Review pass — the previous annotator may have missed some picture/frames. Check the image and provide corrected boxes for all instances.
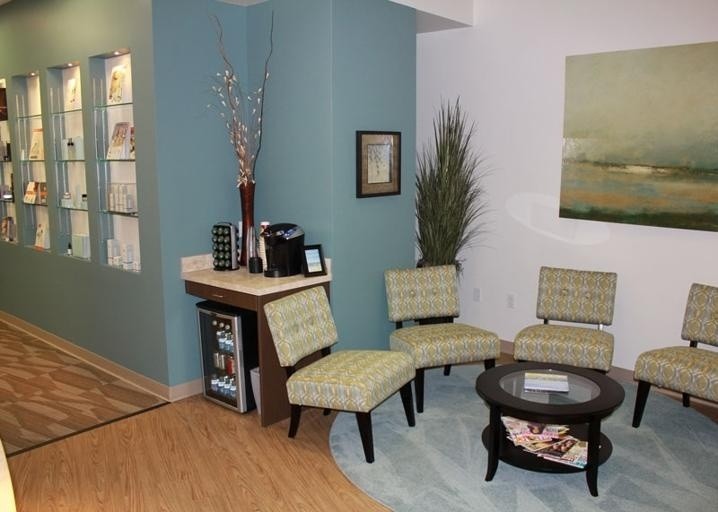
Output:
[301,244,327,277]
[357,130,401,198]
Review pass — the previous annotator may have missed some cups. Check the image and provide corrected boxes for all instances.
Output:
[248,257,263,273]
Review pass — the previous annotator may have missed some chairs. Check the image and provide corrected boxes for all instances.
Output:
[383,266,501,413]
[631,282,718,427]
[264,284,420,462]
[514,268,617,375]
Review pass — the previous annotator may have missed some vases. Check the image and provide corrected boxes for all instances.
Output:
[239,182,257,267]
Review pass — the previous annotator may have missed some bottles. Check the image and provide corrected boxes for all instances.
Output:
[218,377,224,394]
[224,379,230,396]
[211,375,218,391]
[229,335,235,354]
[224,334,232,351]
[230,382,237,399]
[218,332,226,351]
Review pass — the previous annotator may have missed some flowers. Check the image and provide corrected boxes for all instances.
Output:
[198,10,276,188]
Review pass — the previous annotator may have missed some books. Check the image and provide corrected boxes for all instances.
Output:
[501,415,589,470]
[524,372,569,393]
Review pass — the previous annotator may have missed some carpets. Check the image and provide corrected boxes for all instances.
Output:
[329,361,718,512]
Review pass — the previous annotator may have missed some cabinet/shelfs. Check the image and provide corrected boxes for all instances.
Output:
[48,59,95,263]
[1,76,20,247]
[88,49,142,272]
[183,259,333,427]
[17,68,54,254]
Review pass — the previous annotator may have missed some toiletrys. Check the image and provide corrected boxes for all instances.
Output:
[67,138,75,160]
[80,194,87,210]
[117,183,127,211]
[109,192,115,210]
[67,242,73,256]
[127,194,134,214]
[61,192,73,208]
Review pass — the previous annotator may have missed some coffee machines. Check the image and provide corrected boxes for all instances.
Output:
[262,223,304,278]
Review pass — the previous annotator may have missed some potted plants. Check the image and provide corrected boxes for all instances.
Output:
[411,103,493,326]
